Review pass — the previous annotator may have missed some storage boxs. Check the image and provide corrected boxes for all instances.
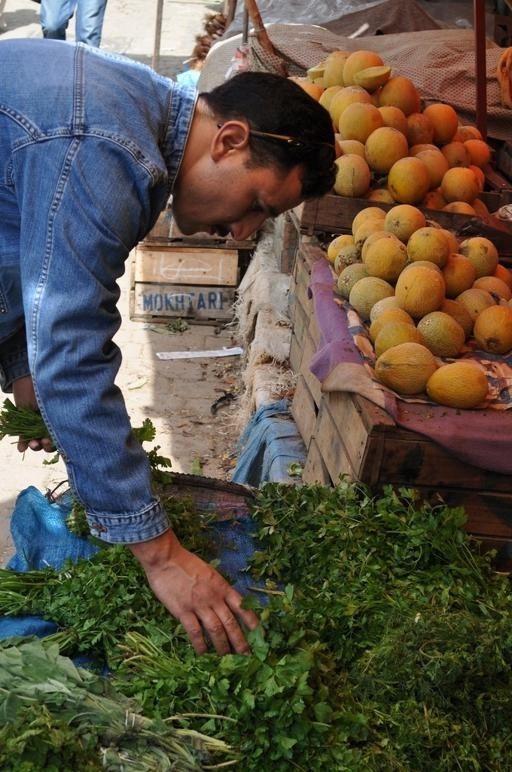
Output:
[128,210,256,326]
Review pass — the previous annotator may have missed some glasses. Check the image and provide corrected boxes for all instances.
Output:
[216,125,336,164]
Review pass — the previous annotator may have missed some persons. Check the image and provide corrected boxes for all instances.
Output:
[0,37,338,659]
[39,0,108,48]
